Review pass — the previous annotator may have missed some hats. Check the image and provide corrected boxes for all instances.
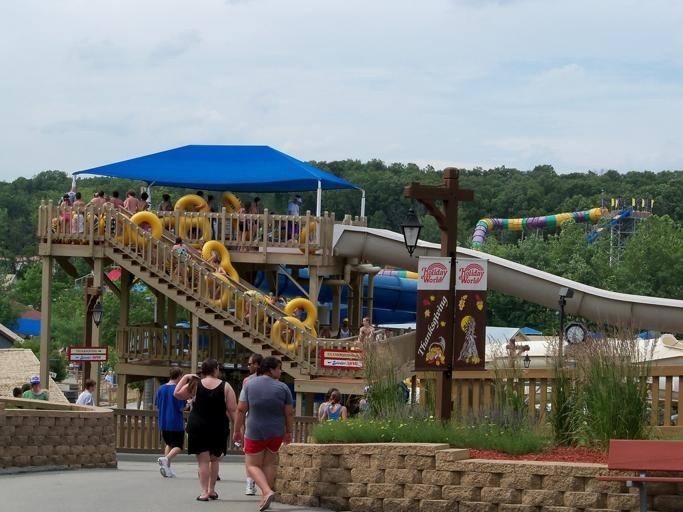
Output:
[30,375,40,384]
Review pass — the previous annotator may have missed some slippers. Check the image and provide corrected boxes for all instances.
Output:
[257,491,274,511]
[196,495,209,501]
[208,491,218,499]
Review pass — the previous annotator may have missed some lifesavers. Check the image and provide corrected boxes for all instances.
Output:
[269,298,316,351]
[222,191,241,212]
[165,254,199,284]
[174,195,212,249]
[199,240,239,308]
[298,222,316,254]
[236,290,266,325]
[50,210,174,247]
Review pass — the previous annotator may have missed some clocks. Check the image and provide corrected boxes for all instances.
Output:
[565,322,587,346]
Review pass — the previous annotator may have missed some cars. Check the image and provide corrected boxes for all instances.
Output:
[642,406,679,426]
[67,364,80,372]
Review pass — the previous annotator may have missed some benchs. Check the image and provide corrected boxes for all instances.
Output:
[596,434,681,512]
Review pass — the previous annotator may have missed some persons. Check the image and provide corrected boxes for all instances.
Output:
[23,376,50,401]
[173,359,238,502]
[13,387,22,399]
[154,367,185,478]
[240,353,264,496]
[22,383,31,394]
[233,356,293,511]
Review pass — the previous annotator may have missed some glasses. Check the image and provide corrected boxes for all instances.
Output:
[248,363,253,367]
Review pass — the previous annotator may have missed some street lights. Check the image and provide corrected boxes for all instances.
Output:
[82,284,103,395]
[555,285,573,367]
[506,338,530,397]
[601,188,604,210]
[401,164,476,428]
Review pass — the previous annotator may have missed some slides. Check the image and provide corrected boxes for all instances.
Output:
[471,208,608,252]
[330,224,682,334]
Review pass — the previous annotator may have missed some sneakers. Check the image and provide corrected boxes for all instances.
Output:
[245,477,257,496]
[158,457,176,478]
